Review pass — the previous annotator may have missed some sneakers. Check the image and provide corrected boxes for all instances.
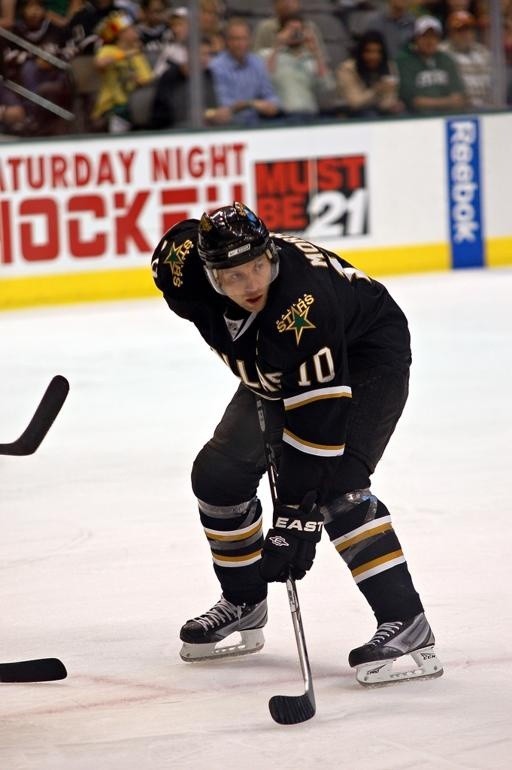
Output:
[180,592,267,644]
[348,611,435,667]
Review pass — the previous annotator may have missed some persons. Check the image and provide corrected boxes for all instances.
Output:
[152,201,445,687]
[0,0,512,143]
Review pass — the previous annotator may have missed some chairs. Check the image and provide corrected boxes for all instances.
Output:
[216,0,415,74]
[70,56,99,133]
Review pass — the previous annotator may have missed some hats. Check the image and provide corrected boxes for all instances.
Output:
[447,11,477,32]
[414,14,444,38]
[95,9,133,44]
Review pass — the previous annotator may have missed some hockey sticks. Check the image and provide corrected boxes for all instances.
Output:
[1,658,68,682]
[0,376,69,456]
[253,390,315,725]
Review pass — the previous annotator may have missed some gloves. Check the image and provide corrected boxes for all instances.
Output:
[258,490,325,582]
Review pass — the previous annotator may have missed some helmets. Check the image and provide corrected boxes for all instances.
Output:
[197,201,281,296]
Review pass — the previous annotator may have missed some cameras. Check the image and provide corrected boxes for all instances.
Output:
[293,29,303,37]
